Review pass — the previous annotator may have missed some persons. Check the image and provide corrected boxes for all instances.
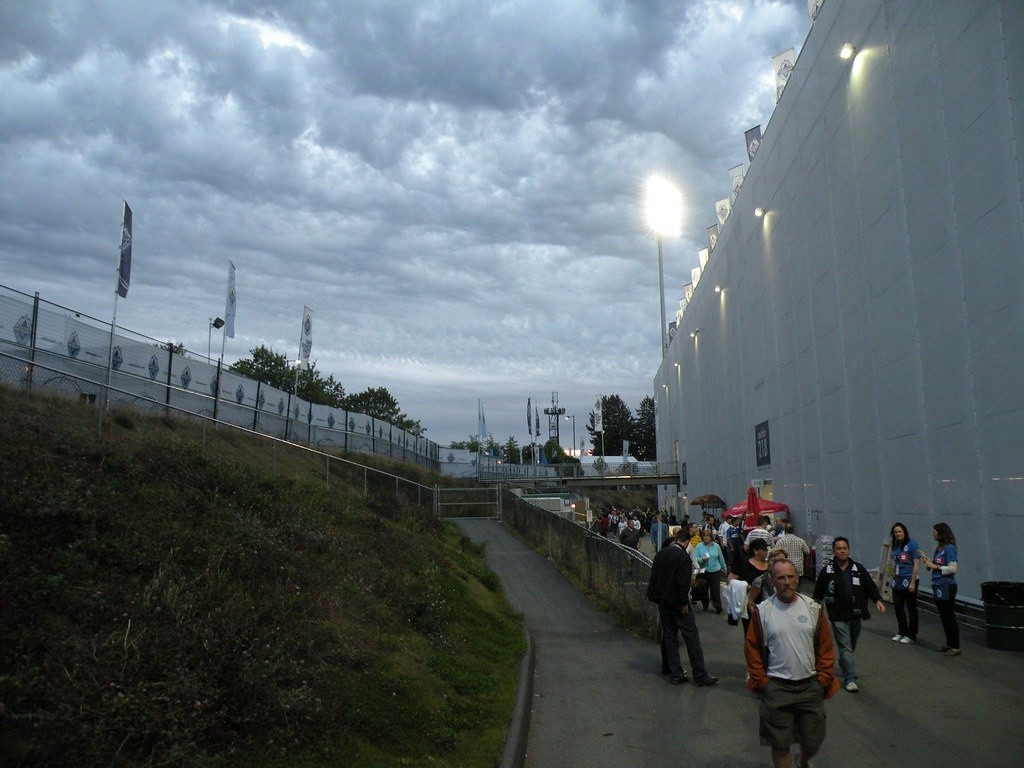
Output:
[888,522,920,644]
[597,505,655,549]
[923,522,960,656]
[652,511,810,639]
[744,558,841,768]
[646,530,720,686]
[812,536,886,691]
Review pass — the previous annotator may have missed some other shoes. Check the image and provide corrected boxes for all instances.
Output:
[891,634,903,642]
[696,674,719,688]
[794,755,810,768]
[900,635,915,644]
[845,681,859,693]
[691,600,697,604]
[671,671,689,684]
[703,605,708,611]
[715,604,724,614]
[943,648,961,656]
[935,644,952,652]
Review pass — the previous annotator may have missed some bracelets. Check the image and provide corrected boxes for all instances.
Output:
[937,565,939,569]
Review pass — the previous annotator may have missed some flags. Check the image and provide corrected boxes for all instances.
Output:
[593,395,602,431]
[526,399,541,436]
[478,400,487,438]
[117,201,133,298]
[224,263,237,339]
[301,306,313,358]
[623,440,629,462]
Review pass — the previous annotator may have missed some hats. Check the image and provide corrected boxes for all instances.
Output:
[749,538,771,548]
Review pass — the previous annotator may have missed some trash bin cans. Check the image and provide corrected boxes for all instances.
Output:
[981,581,1024,650]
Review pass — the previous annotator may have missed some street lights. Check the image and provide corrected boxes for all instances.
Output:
[565,414,576,456]
[207,316,225,365]
[600,429,606,463]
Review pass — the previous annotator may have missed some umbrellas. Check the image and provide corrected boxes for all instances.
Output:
[690,493,726,508]
[721,497,788,518]
[744,486,760,530]
